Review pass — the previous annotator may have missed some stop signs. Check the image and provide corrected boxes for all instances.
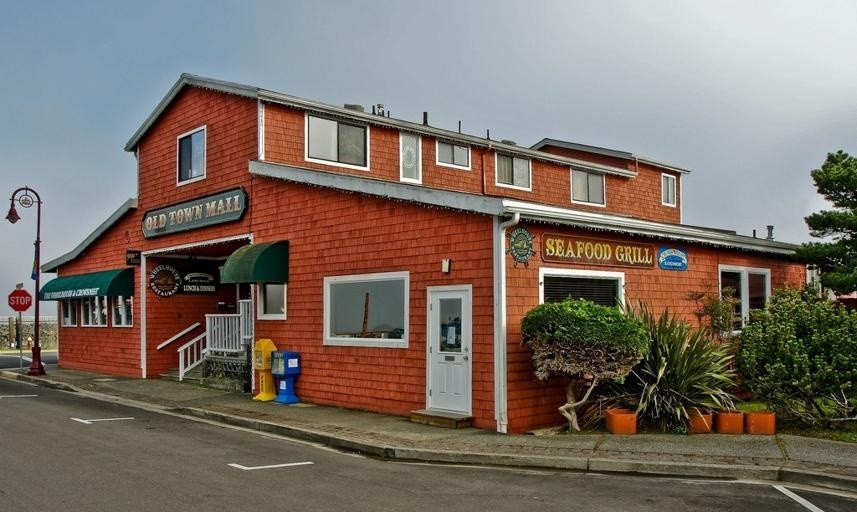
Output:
[8,289,31,312]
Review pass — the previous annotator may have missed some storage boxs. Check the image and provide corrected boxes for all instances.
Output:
[744,410,777,436]
[713,409,743,435]
[684,407,713,433]
[605,407,636,435]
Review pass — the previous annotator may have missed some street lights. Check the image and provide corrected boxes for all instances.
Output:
[4,185,47,376]
